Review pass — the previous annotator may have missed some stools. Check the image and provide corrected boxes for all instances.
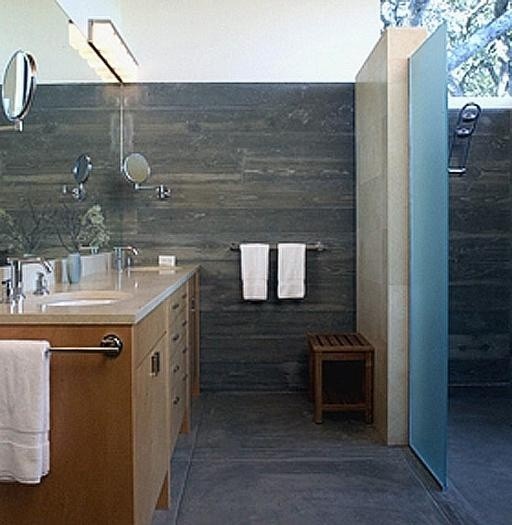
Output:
[307,331,374,425]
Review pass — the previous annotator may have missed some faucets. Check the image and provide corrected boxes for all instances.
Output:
[1,256,53,304]
[112,246,139,273]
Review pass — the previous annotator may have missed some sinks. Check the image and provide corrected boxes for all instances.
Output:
[34,288,132,308]
[125,264,182,273]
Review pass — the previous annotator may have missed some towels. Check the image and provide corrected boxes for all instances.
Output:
[241,243,269,301]
[277,242,306,299]
[0,340,50,484]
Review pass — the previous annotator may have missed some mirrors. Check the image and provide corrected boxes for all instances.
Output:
[122,151,170,200]
[0,49,37,131]
[0,0,126,292]
[73,152,92,183]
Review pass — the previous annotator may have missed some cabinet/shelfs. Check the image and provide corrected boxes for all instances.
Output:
[0,264,199,525]
